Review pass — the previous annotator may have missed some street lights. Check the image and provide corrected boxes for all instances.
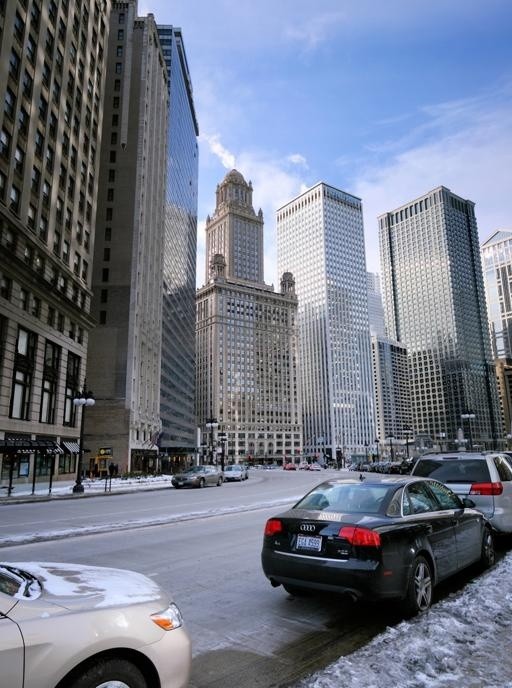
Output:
[461,404,476,451]
[72,378,95,491]
[402,424,413,458]
[205,417,219,464]
[386,429,395,460]
[374,436,380,463]
[364,440,369,461]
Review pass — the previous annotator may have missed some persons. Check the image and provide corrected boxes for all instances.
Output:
[109,462,115,479]
[114,463,118,475]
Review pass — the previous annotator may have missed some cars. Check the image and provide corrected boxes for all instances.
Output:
[283,461,321,470]
[0,560,190,688]
[262,470,505,611]
[408,450,512,542]
[171,464,224,488]
[223,464,248,481]
[348,456,415,475]
